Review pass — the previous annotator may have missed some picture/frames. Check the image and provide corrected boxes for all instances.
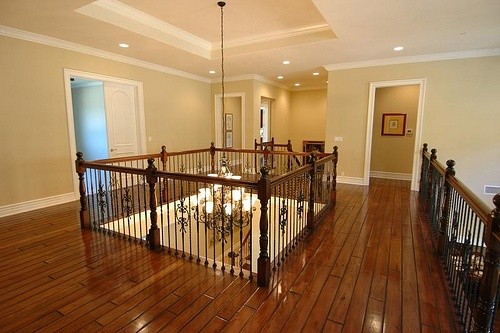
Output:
[381,112,407,137]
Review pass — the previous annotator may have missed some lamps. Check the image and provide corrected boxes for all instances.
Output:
[192,0,259,240]
